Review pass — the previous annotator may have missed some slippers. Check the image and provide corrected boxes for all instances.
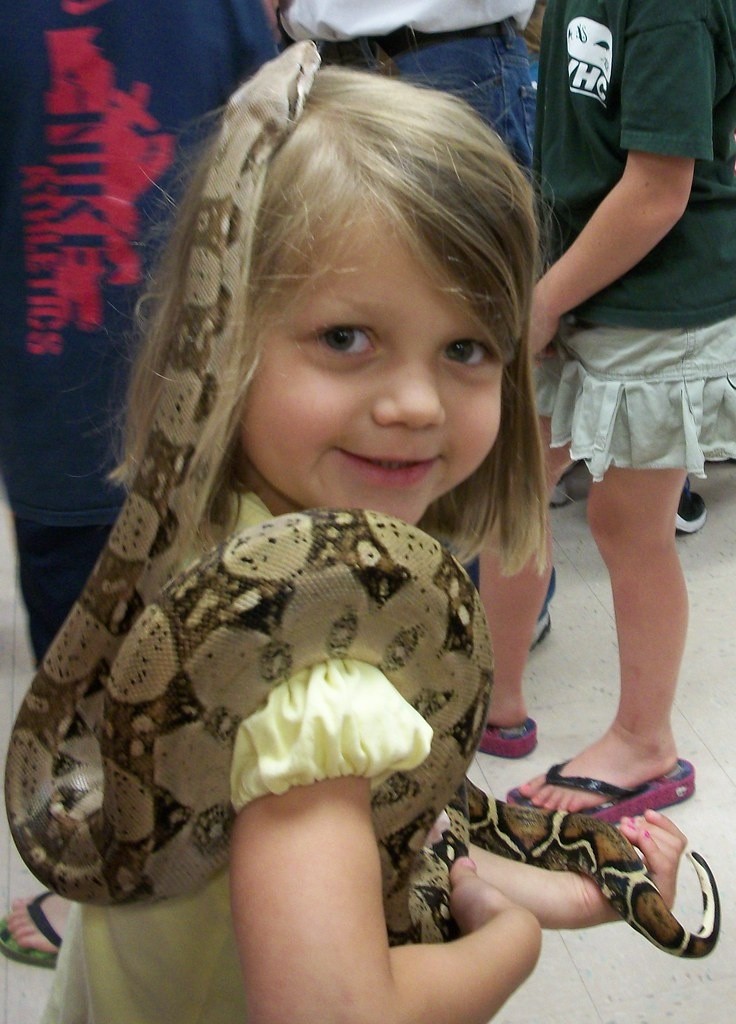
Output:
[0,891,63,969]
[507,758,697,825]
[478,717,538,759]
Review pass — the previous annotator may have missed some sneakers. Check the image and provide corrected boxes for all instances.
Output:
[528,565,556,651]
[675,476,707,533]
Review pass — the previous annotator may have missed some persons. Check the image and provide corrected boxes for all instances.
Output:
[44,40,691,1024]
[275,0,557,651]
[476,0,736,824]
[0,0,279,671]
